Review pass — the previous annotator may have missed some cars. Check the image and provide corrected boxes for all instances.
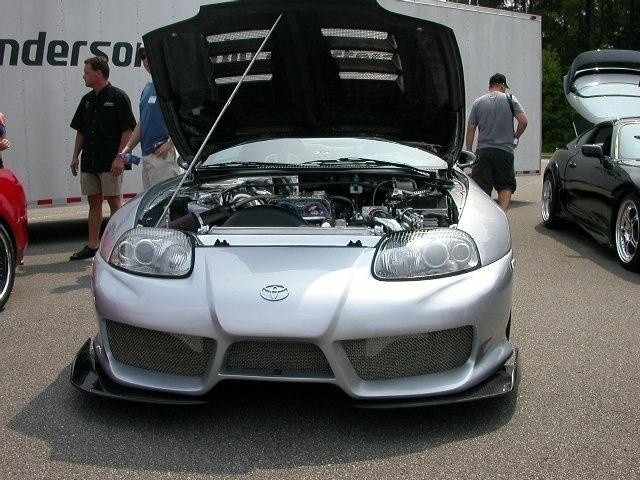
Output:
[0,167,30,311]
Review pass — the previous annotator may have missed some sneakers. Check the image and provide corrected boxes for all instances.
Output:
[70,245,98,261]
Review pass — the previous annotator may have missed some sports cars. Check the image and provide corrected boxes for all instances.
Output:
[68,0,522,414]
[541,47,640,271]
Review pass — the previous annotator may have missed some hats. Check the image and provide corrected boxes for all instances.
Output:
[490,73,510,89]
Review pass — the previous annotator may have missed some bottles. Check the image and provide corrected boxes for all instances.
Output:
[116,152,144,168]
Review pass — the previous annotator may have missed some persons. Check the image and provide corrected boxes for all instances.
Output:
[69,56,136,262]
[465,73,528,213]
[0,112,12,168]
[117,43,187,193]
